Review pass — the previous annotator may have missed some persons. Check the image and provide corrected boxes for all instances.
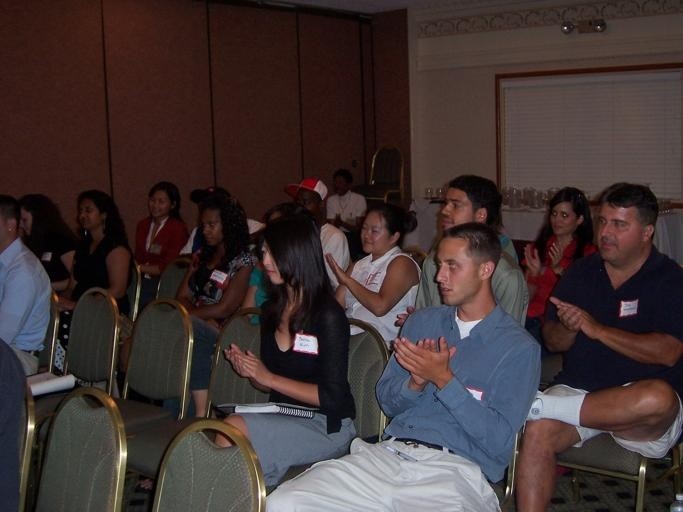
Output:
[324,170,368,264]
[134,180,190,318]
[285,176,350,294]
[213,213,357,486]
[325,200,426,356]
[520,186,599,357]
[394,174,529,331]
[239,203,289,328]
[177,186,267,267]
[163,197,260,418]
[512,182,683,512]
[266,222,541,512]
[19,192,78,292]
[52,190,133,394]
[0,193,51,377]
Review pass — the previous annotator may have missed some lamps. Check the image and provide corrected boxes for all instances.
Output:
[561,19,607,34]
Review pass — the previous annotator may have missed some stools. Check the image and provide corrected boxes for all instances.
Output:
[505,377,679,512]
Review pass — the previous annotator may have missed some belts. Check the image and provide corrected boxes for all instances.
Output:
[385,436,454,453]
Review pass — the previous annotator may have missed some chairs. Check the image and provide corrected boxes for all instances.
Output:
[37,386,128,512]
[25,290,60,378]
[266,317,389,496]
[156,258,192,300]
[17,382,36,512]
[153,419,266,512]
[33,287,120,429]
[37,298,194,477]
[127,258,142,322]
[124,307,270,480]
[365,143,404,204]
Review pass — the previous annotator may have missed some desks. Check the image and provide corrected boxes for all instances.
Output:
[402,198,672,260]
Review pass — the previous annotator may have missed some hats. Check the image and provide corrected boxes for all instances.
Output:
[190,186,230,203]
[285,177,328,200]
